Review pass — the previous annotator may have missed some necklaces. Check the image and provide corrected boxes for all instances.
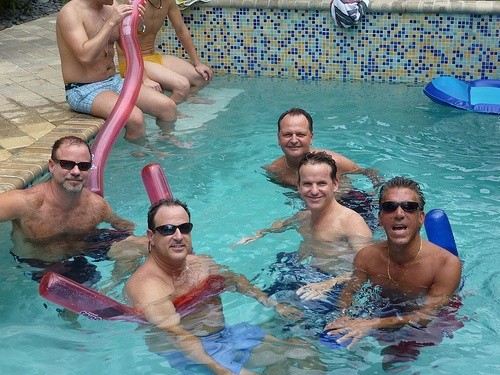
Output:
[387,238,422,285]
[148,0,162,9]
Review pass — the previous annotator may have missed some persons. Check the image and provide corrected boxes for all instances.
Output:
[115,0,215,102]
[294,152,372,236]
[264,109,357,183]
[352,176,462,297]
[123,198,217,299]
[0,136,147,246]
[56,0,191,140]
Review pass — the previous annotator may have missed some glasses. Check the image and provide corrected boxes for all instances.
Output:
[152,223,193,236]
[53,159,91,171]
[379,201,422,212]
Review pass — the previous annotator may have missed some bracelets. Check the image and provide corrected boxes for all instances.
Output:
[138,24,146,33]
